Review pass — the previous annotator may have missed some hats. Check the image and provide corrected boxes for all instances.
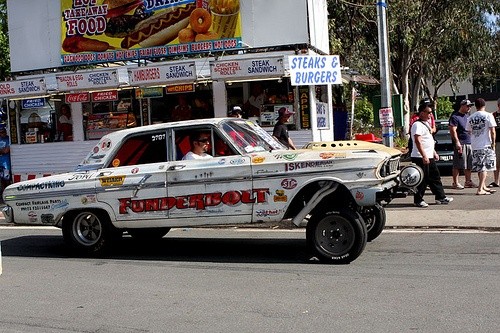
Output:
[420,98,430,104]
[461,99,474,106]
[232,106,242,113]
[275,106,296,121]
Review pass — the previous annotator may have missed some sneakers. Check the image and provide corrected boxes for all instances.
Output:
[414,201,429,208]
[464,181,479,188]
[436,197,453,204]
[452,182,464,189]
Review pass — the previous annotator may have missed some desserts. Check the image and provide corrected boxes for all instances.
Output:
[105,11,154,38]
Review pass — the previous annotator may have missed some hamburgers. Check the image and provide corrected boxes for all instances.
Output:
[101,0,144,17]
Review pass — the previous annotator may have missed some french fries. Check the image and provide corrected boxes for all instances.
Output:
[208,0,241,40]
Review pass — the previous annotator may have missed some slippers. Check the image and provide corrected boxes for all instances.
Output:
[476,190,491,195]
[487,189,497,193]
[488,182,499,187]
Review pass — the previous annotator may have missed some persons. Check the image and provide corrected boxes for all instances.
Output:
[182,132,211,161]
[172,95,210,122]
[465,98,497,195]
[272,107,296,150]
[410,103,453,208]
[487,98,500,188]
[0,127,11,192]
[232,106,242,118]
[55,104,72,142]
[409,98,437,135]
[448,99,479,190]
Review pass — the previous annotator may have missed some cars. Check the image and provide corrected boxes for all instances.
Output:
[407,119,466,176]
[4,118,425,266]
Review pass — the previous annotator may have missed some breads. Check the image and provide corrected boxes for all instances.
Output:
[179,29,220,44]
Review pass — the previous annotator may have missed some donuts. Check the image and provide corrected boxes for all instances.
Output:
[189,8,212,33]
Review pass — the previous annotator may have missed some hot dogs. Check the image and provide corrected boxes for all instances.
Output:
[121,4,196,48]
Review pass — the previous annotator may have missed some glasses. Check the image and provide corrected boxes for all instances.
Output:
[197,138,210,142]
[423,111,433,114]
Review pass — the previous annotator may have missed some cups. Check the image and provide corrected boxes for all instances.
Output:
[209,11,240,40]
[41,135,44,143]
